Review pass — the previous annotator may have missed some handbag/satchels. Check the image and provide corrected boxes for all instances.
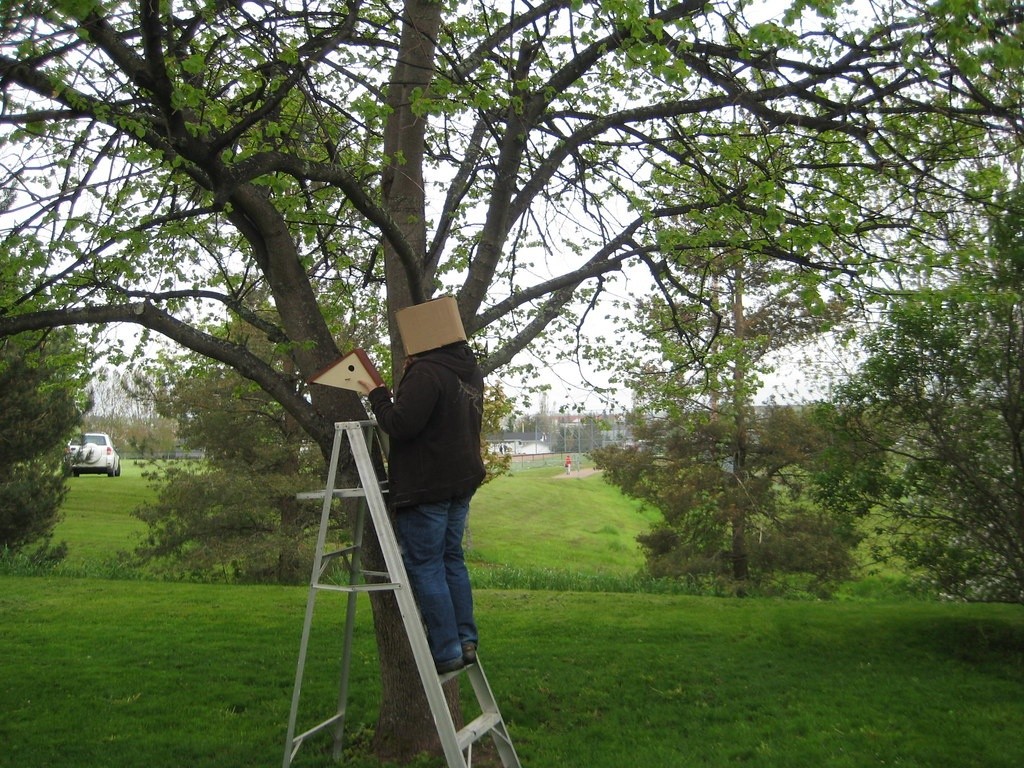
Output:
[565,464,568,467]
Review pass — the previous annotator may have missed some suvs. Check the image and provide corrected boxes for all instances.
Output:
[67,432,120,478]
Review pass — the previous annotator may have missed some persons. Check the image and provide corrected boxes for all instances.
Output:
[566,455,572,475]
[358,341,486,674]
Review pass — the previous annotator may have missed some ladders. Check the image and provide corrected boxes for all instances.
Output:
[282,419,523,768]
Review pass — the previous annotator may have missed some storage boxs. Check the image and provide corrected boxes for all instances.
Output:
[393,294,468,357]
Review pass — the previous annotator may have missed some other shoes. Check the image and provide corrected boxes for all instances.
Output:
[464,641,477,664]
[417,658,463,674]
